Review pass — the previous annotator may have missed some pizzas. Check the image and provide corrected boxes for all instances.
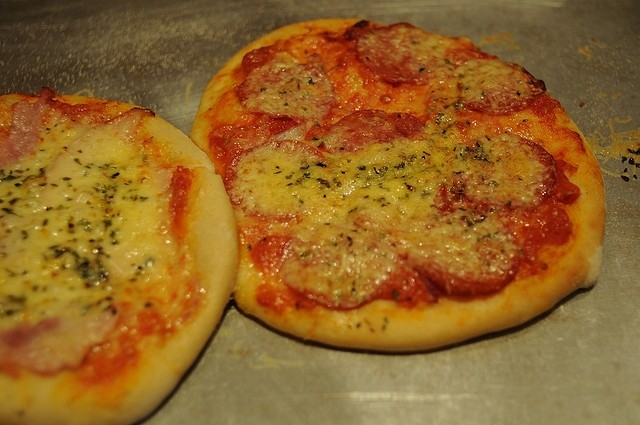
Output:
[190,16,606,357]
[0,87,239,424]
